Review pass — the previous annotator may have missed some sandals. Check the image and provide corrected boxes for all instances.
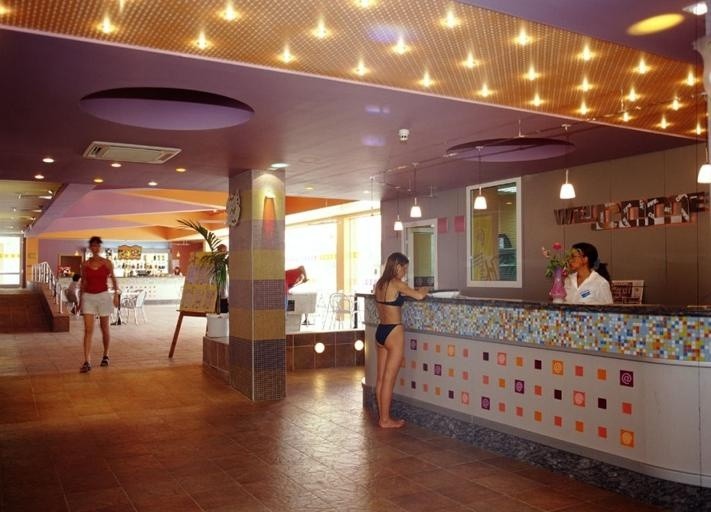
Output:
[79,362,91,373]
[100,356,108,366]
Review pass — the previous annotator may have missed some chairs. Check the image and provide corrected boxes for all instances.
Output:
[116,288,149,325]
[328,291,360,328]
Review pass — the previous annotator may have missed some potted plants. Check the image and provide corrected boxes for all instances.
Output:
[176,218,229,338]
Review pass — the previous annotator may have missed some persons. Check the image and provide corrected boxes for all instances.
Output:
[563,242,617,306]
[75,235,122,373]
[286,266,309,295]
[65,274,82,314]
[216,244,230,314]
[372,252,433,428]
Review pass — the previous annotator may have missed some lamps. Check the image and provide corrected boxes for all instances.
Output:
[394,123,576,232]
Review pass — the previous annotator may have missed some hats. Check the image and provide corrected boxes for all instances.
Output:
[88,237,103,245]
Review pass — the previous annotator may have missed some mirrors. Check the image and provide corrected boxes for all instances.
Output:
[467,176,522,288]
